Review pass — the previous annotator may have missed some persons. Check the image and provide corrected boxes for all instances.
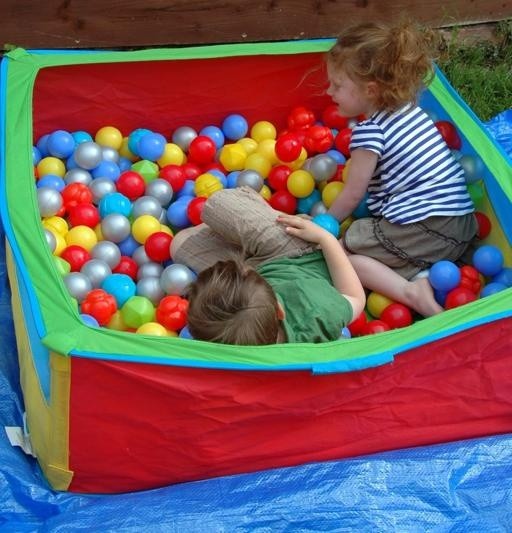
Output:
[169,186,366,345]
[295,21,479,320]
